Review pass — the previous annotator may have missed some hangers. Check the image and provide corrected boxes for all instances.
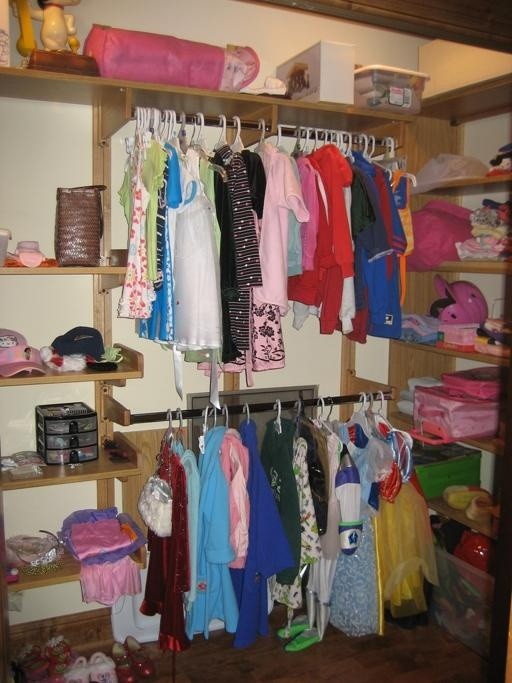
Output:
[166,391,411,480]
[124,106,417,188]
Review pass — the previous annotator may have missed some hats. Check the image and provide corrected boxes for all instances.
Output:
[0,327,47,378]
[51,326,105,356]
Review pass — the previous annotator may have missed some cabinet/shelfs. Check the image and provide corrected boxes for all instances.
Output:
[1,66,511,654]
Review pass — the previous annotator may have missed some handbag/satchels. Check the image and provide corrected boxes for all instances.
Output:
[136,433,174,539]
[54,185,108,266]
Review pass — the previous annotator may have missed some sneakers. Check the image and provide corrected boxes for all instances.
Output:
[275,614,324,654]
[88,651,119,683]
[441,483,492,510]
[463,494,494,522]
[11,635,73,683]
[63,655,91,683]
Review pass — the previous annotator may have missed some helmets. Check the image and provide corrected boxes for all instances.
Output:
[429,273,488,325]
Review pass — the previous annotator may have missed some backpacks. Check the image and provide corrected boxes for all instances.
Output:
[404,365,502,447]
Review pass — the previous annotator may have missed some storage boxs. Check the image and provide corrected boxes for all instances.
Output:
[276,40,356,105]
[432,545,496,661]
[354,64,430,115]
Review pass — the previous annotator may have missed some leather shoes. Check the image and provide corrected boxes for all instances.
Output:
[110,640,137,683]
[123,635,156,679]
